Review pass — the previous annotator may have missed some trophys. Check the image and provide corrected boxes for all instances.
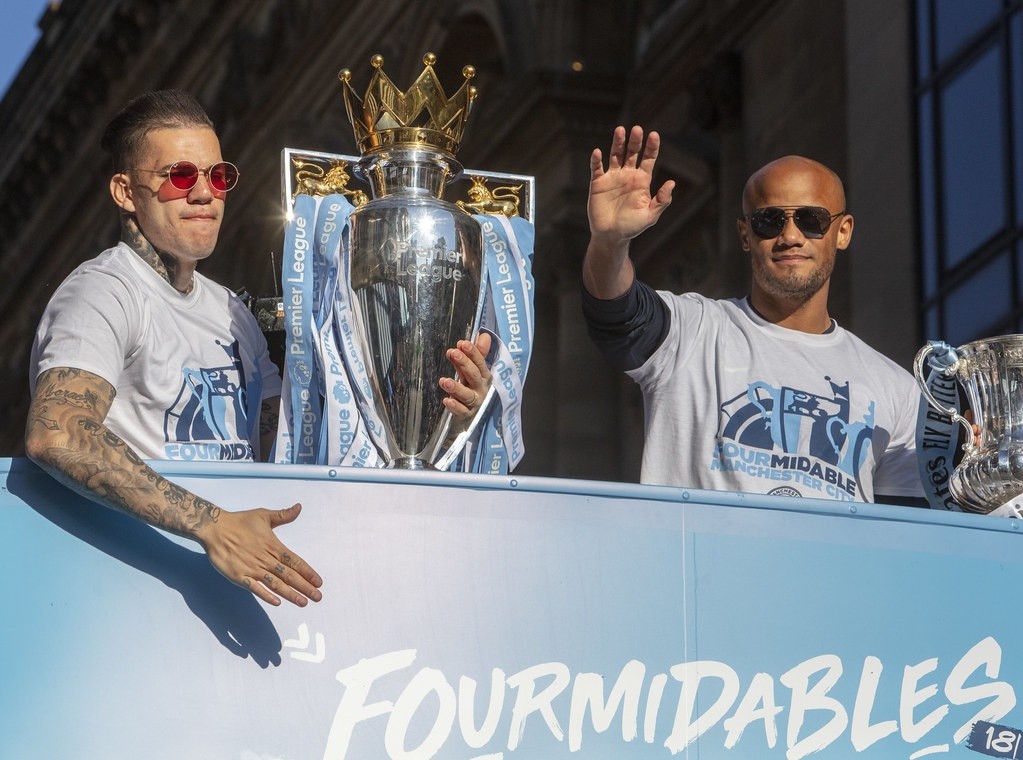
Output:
[283,51,521,470]
[913,334,1023,520]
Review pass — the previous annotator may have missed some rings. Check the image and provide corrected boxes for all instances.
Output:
[465,392,478,406]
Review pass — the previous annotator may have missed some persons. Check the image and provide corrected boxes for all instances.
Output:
[23,86,493,607]
[582,125,982,510]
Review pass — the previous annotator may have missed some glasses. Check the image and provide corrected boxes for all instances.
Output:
[741,204,845,239]
[121,160,241,193]
